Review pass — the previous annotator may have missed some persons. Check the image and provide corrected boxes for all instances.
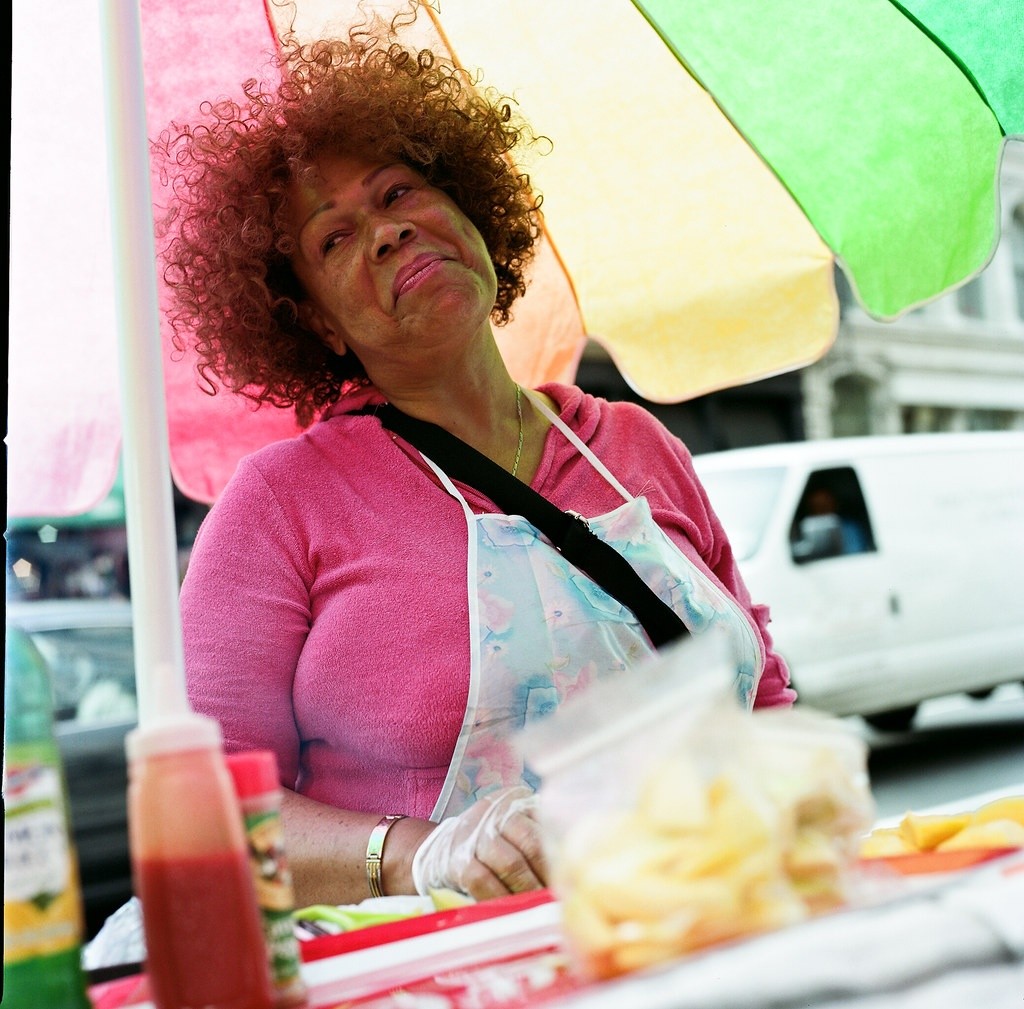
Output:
[152,23,799,905]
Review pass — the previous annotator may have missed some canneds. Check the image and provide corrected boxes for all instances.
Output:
[224,749,312,1009]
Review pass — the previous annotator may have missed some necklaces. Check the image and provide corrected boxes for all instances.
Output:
[511,383,523,476]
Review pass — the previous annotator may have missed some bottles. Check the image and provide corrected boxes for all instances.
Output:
[0,620,94,1009]
[124,711,311,1009]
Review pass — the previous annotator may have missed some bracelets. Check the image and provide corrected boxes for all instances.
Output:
[364,814,409,899]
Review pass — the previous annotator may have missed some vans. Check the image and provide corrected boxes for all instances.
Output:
[688,426,1024,745]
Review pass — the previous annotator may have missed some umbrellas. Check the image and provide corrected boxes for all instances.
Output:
[4,1,1022,730]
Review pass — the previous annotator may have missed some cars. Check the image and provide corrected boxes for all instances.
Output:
[1,603,139,944]
